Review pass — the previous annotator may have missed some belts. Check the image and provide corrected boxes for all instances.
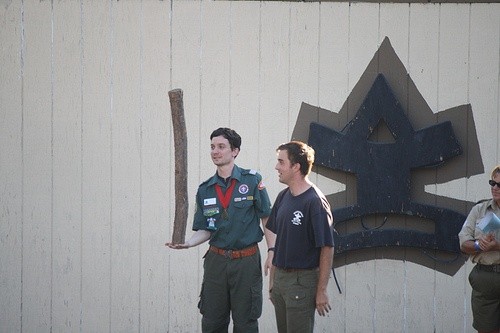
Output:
[286,267,313,271]
[209,245,258,258]
[478,263,500,273]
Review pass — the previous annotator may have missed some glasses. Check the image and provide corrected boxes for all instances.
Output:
[489,179,500,186]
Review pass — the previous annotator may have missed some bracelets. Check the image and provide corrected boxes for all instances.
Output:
[268,248,275,251]
[475,241,480,249]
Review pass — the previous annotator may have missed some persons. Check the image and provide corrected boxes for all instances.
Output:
[458,166,500,333]
[166,128,276,333]
[266,141,335,333]
